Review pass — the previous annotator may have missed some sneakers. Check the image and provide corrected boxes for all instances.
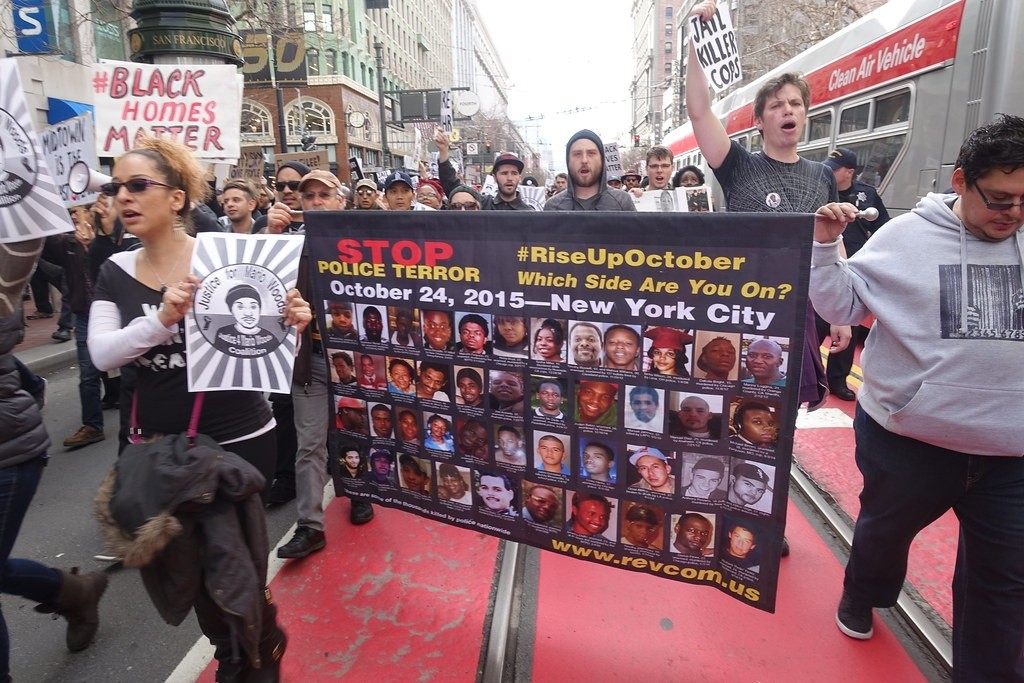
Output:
[835,587,873,639]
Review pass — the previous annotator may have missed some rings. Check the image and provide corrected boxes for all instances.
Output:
[179,282,183,289]
[305,309,306,313]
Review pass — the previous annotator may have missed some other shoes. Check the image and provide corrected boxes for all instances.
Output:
[51,326,71,340]
[26,309,53,319]
[827,383,856,401]
[62,425,107,446]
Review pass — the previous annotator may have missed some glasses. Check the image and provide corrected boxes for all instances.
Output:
[648,163,670,169]
[274,180,300,191]
[357,190,373,196]
[530,494,559,512]
[101,178,175,195]
[450,201,478,210]
[969,174,1024,210]
[626,179,637,182]
[416,193,437,198]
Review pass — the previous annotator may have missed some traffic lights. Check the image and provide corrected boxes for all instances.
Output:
[635,135,640,146]
[485,142,492,153]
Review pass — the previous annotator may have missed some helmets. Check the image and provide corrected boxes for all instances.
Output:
[369,447,394,461]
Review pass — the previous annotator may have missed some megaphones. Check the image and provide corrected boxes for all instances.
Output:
[67,161,112,194]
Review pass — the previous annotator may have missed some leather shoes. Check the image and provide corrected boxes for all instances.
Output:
[351,497,374,523]
[276,527,326,558]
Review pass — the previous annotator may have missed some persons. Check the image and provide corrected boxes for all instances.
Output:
[22,194,139,448]
[0,302,108,683]
[523,147,705,198]
[87,142,312,683]
[815,148,889,401]
[338,161,485,210]
[190,162,312,234]
[325,298,789,573]
[543,128,637,211]
[808,112,1024,683]
[685,0,853,557]
[435,127,533,209]
[261,169,373,558]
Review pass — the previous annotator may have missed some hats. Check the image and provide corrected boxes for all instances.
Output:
[620,168,641,183]
[448,186,480,202]
[275,161,311,179]
[491,154,524,174]
[644,328,694,352]
[627,505,659,524]
[298,169,342,188]
[734,463,773,492]
[820,148,857,169]
[607,176,621,182]
[521,177,538,187]
[385,173,413,190]
[399,455,430,478]
[324,300,353,314]
[355,179,376,192]
[696,340,708,373]
[338,397,366,412]
[630,447,669,466]
[456,368,483,393]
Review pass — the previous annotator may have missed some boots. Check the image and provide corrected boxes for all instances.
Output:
[33,568,108,654]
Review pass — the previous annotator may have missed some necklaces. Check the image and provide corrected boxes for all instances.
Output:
[145,256,179,291]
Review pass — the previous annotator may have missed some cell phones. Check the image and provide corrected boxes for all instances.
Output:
[289,211,305,222]
